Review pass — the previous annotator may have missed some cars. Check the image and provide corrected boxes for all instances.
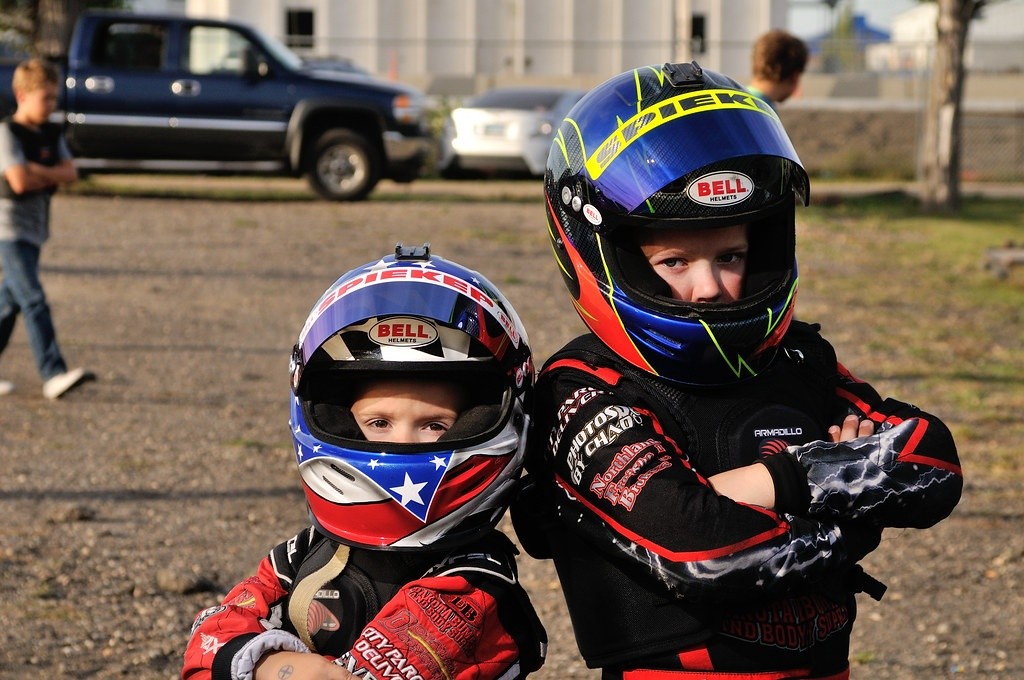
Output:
[443,87,583,180]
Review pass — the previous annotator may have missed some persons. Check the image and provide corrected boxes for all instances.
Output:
[0,57,85,401]
[507,62,963,680]
[178,242,549,680]
[745,31,810,107]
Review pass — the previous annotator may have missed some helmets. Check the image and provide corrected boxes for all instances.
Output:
[287,243,536,551]
[545,62,814,399]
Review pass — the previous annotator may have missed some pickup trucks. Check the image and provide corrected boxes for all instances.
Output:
[0,9,456,203]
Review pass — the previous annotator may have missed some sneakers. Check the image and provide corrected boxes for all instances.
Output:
[0,381,16,396]
[42,367,85,400]
